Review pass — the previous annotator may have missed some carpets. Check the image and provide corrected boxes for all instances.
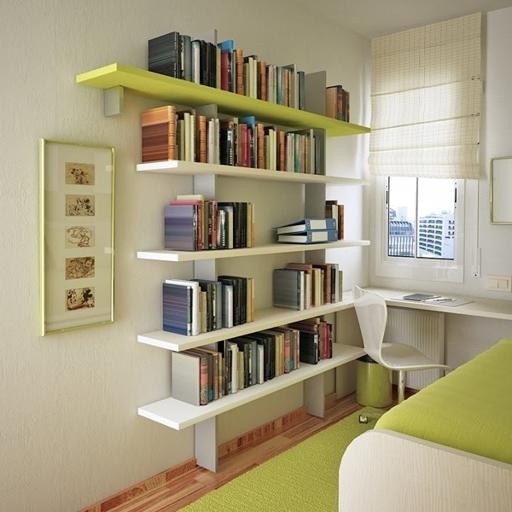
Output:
[174,406,391,512]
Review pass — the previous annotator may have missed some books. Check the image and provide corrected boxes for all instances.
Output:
[149,31,306,112]
[217,314,334,400]
[272,263,344,311]
[173,348,219,406]
[162,278,215,335]
[327,200,345,241]
[221,116,324,175]
[277,231,337,244]
[218,202,257,251]
[326,84,347,120]
[140,106,219,165]
[164,193,217,252]
[403,293,434,302]
[217,276,256,331]
[273,218,337,233]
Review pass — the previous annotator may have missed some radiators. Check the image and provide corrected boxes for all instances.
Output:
[383,307,445,392]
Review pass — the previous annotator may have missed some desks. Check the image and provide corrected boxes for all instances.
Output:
[365,286,512,321]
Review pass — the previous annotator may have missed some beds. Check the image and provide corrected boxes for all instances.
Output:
[335,334,512,512]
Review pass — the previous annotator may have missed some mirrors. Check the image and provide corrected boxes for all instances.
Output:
[490,156,511,225]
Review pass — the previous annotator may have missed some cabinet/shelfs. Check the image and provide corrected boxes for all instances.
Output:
[75,62,371,476]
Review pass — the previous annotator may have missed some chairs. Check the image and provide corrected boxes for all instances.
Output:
[349,283,449,409]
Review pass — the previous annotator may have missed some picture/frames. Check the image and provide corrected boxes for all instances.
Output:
[38,137,119,336]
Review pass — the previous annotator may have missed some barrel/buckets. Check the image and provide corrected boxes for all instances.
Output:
[354,354,393,408]
